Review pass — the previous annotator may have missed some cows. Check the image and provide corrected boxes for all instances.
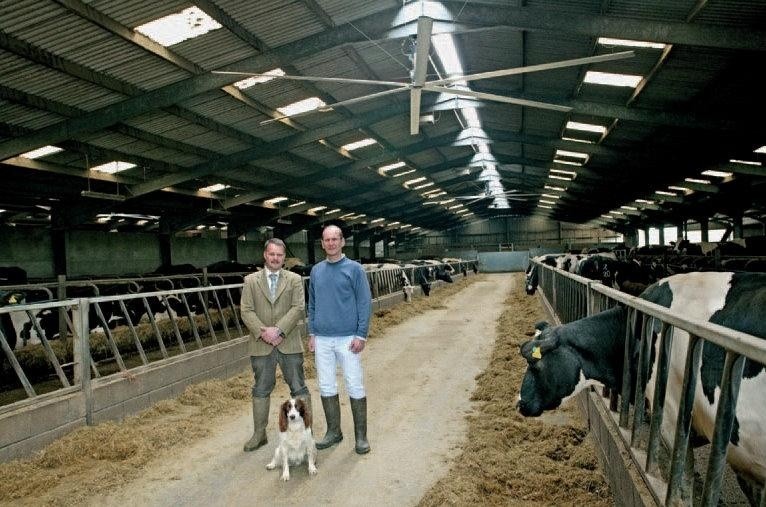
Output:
[1,256,480,355]
[516,234,765,506]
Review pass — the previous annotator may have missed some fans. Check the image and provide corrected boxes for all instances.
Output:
[209,16,637,136]
[441,178,557,209]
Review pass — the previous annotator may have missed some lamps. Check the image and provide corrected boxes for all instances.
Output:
[205,184,232,217]
[275,200,294,226]
[79,153,126,202]
[385,0,516,209]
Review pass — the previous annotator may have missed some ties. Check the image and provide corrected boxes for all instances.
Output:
[270,274,277,298]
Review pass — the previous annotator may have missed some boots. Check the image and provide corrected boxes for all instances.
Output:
[293,394,314,436]
[244,397,271,452]
[315,393,344,449]
[350,395,372,454]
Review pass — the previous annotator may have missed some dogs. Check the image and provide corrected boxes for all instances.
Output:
[264,395,319,482]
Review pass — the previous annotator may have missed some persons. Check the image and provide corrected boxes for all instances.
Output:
[241,238,312,452]
[307,225,371,454]
